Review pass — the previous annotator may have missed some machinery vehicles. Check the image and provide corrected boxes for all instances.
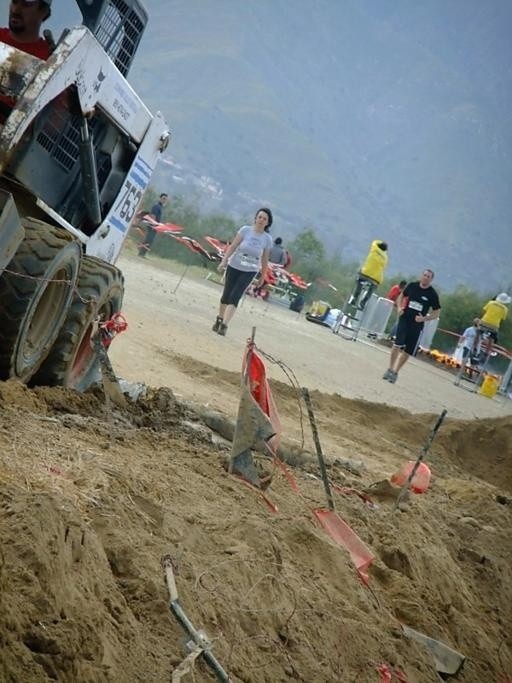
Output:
[1,1,177,397]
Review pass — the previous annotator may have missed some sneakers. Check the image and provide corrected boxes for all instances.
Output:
[360,300,365,308]
[384,368,397,384]
[213,316,228,336]
[351,300,356,305]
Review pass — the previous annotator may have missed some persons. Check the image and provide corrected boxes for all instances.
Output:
[0,0,72,135]
[473,292,512,363]
[386,280,406,303]
[282,250,292,270]
[382,270,440,384]
[212,207,272,336]
[348,240,388,310]
[457,318,481,380]
[138,193,167,259]
[257,237,286,300]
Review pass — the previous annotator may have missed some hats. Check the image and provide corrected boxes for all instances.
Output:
[496,292,512,304]
[472,318,480,325]
